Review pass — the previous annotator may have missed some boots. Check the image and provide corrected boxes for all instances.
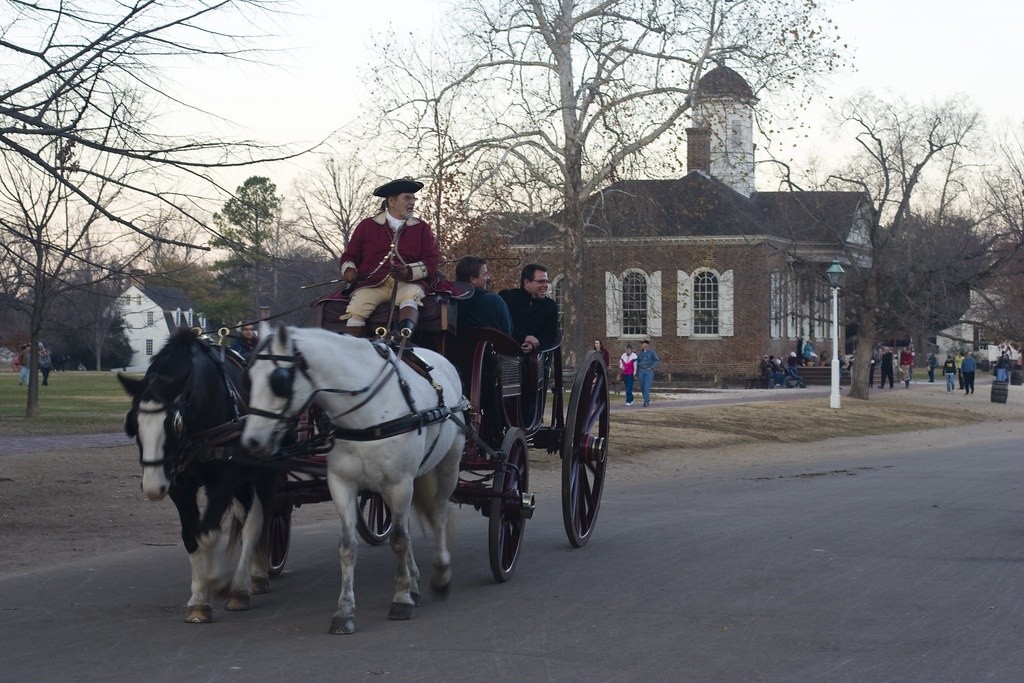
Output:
[398,306,420,341]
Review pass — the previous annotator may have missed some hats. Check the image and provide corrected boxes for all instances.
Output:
[639,339,649,344]
[791,352,796,357]
[373,179,424,198]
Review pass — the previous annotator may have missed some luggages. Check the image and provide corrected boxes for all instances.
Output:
[991,380,1009,404]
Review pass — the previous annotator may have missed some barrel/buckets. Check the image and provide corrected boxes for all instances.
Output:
[981,360,989,372]
[990,380,1008,404]
[1010,370,1023,385]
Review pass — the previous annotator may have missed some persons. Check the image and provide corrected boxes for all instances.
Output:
[498,264,558,353]
[456,255,513,337]
[18,342,53,387]
[341,180,440,342]
[636,339,659,407]
[760,353,806,389]
[620,344,638,406]
[591,339,609,409]
[839,346,1009,395]
[796,336,818,366]
[230,321,258,358]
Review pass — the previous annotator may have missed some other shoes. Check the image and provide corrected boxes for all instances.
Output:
[971,388,975,394]
[625,400,634,407]
[878,385,883,389]
[643,402,649,407]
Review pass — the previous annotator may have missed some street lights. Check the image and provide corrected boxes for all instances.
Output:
[826,256,846,409]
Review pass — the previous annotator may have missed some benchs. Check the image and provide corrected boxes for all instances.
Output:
[526,328,565,428]
[744,378,768,388]
[315,294,458,357]
[461,326,523,431]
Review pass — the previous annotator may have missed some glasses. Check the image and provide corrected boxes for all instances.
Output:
[533,279,550,284]
[402,197,419,202]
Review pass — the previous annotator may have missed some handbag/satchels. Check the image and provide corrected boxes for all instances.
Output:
[926,364,931,373]
[616,373,624,381]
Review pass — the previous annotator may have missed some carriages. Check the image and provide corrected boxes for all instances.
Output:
[114,281,610,636]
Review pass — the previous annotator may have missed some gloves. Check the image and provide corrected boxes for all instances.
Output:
[345,268,358,284]
[390,263,413,282]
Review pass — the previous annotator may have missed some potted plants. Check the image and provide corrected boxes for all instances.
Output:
[819,351,829,366]
[805,357,815,367]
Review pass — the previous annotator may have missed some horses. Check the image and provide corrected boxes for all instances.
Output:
[116,321,466,636]
